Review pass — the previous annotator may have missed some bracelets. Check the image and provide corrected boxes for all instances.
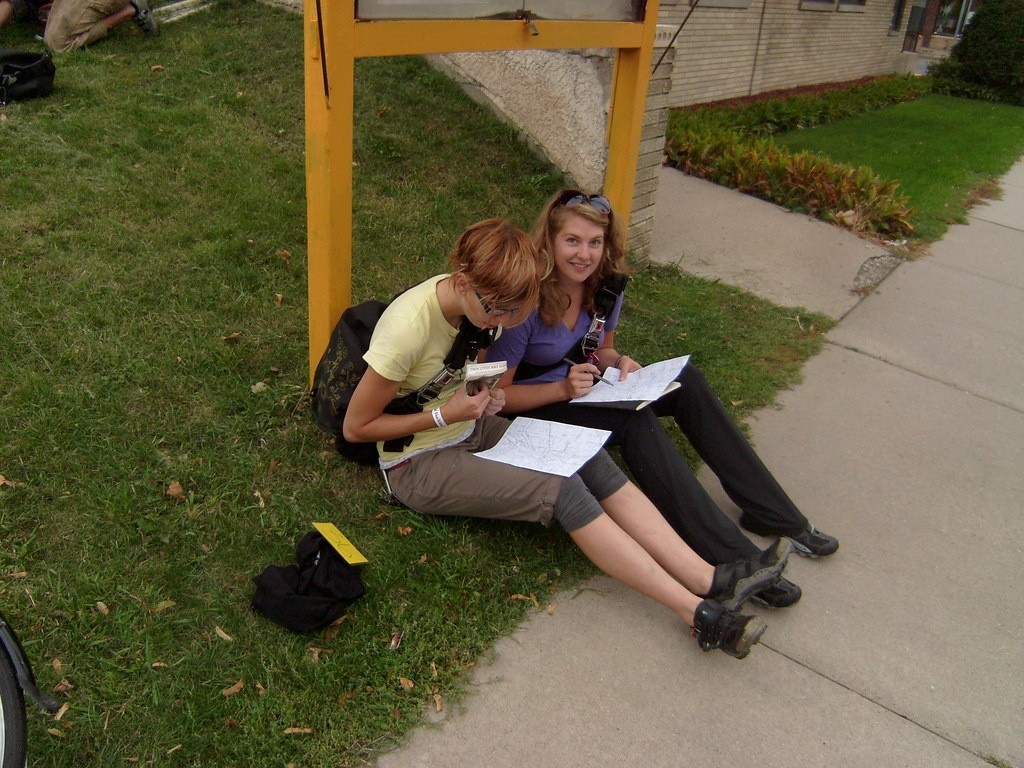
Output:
[431,407,448,429]
[615,355,630,368]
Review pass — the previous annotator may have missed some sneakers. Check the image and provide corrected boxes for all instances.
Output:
[747,576,801,611]
[131,0,159,37]
[689,600,769,659]
[712,535,792,610]
[781,522,840,556]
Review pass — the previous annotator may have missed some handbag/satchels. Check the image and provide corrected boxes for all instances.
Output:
[305,285,479,435]
[0,50,55,106]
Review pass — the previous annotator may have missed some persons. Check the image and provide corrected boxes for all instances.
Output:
[342,216,792,660]
[485,187,839,611]
[0,0,161,56]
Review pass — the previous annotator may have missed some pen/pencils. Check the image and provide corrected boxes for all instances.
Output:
[564,357,614,387]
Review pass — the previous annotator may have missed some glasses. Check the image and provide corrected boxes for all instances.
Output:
[557,190,612,216]
[469,283,522,320]
